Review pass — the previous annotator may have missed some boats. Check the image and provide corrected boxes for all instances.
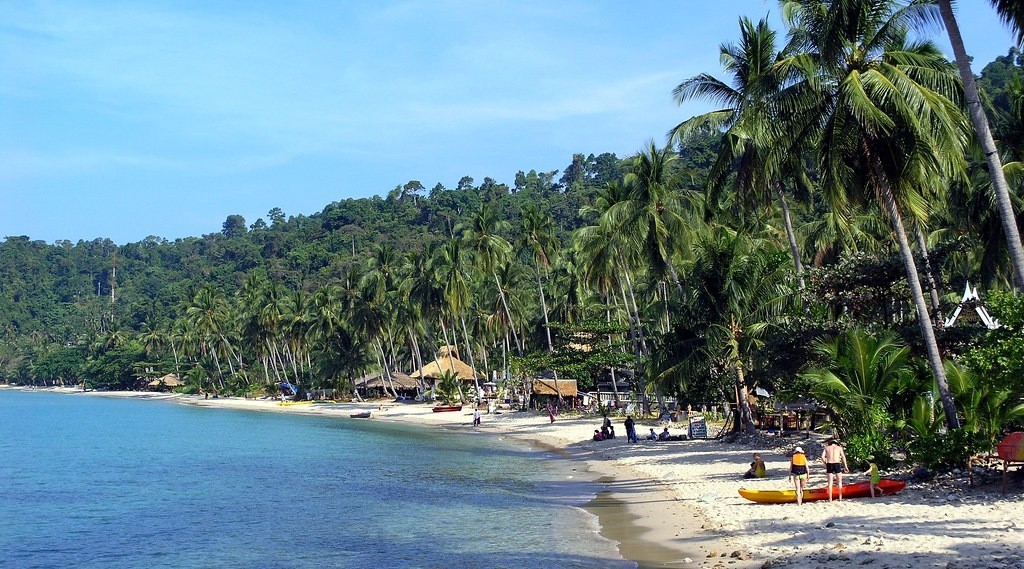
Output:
[350,412,371,418]
[431,406,462,412]
[278,400,336,407]
[737,478,906,503]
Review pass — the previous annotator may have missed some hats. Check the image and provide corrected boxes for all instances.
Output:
[866,455,875,461]
[793,447,804,453]
[828,439,840,445]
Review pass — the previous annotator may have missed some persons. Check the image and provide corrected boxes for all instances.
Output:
[647,428,669,441]
[624,415,635,443]
[789,447,809,508]
[473,409,480,426]
[744,453,765,479]
[822,440,849,502]
[862,455,883,498]
[593,413,616,440]
